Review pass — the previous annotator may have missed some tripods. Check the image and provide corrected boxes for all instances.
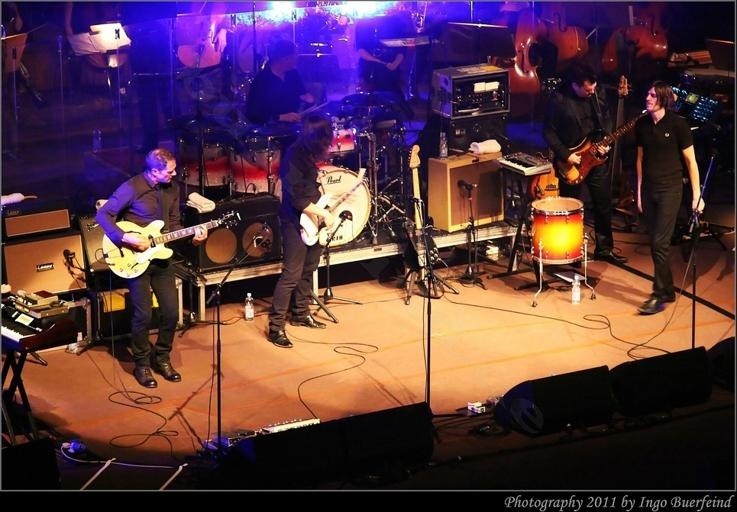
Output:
[313,219,363,315]
[2,44,145,164]
[178,270,223,338]
[353,109,413,244]
[443,190,487,290]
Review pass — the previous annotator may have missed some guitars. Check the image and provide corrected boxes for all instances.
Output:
[401,144,440,272]
[102,210,242,280]
[553,107,650,186]
[299,182,362,246]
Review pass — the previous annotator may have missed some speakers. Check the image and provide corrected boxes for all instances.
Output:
[204,420,348,489]
[340,402,434,489]
[73,210,129,290]
[2,230,88,298]
[494,366,611,439]
[609,347,712,417]
[708,337,735,393]
[4,208,72,240]
[428,151,505,233]
[192,191,282,274]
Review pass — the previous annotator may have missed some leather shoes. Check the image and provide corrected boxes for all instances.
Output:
[155,360,181,382]
[594,249,628,263]
[637,298,663,314]
[291,314,326,329]
[268,330,293,348]
[644,292,676,304]
[134,368,157,388]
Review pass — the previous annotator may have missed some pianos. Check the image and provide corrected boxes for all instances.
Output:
[495,151,551,176]
[0,303,56,353]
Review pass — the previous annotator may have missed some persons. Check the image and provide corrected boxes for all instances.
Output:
[1,1,685,134]
[93,148,208,390]
[264,113,334,348]
[545,67,630,266]
[635,78,704,314]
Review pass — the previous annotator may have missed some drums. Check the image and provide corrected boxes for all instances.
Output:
[274,158,372,248]
[172,111,360,194]
[530,197,584,266]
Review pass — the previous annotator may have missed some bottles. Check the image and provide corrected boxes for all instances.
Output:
[76,332,83,347]
[244,293,254,321]
